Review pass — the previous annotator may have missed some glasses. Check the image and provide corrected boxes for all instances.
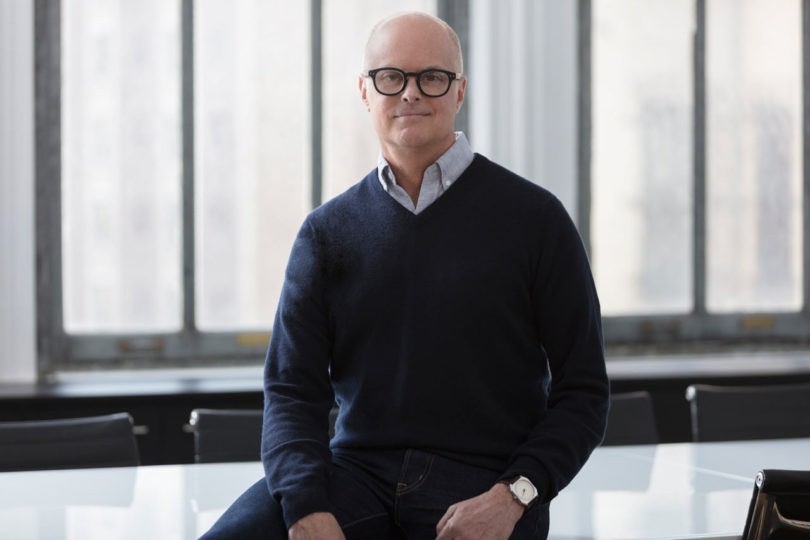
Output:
[362,66,462,98]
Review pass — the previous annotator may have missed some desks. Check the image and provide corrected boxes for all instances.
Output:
[0,347,810,420]
[0,438,810,540]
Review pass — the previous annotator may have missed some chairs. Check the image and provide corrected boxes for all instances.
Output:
[190,409,264,462]
[601,392,658,446]
[686,384,810,442]
[0,412,142,470]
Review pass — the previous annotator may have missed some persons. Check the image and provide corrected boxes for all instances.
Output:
[198,13,610,540]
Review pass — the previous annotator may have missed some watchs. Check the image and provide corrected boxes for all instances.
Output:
[497,475,539,511]
